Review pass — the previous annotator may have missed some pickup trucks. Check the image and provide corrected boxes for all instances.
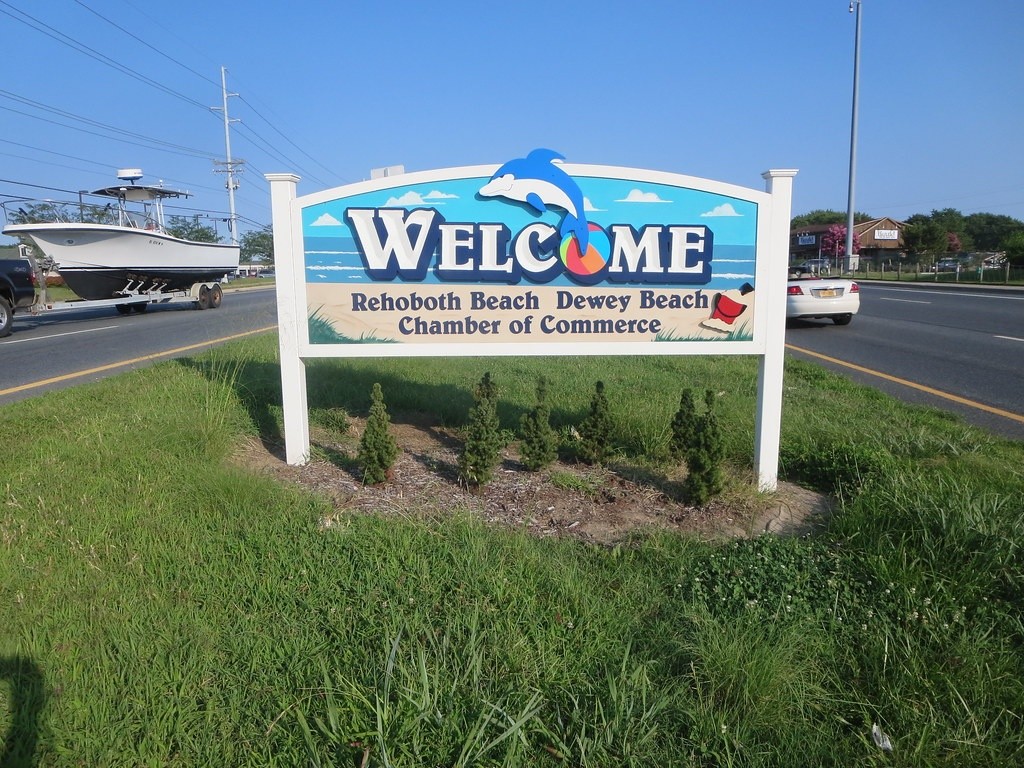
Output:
[0,256,40,338]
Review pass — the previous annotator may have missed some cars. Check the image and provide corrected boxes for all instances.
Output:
[788,258,831,276]
[255,269,275,278]
[787,266,860,324]
[955,253,978,267]
[932,259,963,271]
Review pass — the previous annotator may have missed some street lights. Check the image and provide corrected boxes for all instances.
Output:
[843,0,861,274]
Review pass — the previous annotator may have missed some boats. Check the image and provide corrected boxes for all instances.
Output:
[0,169,241,300]
